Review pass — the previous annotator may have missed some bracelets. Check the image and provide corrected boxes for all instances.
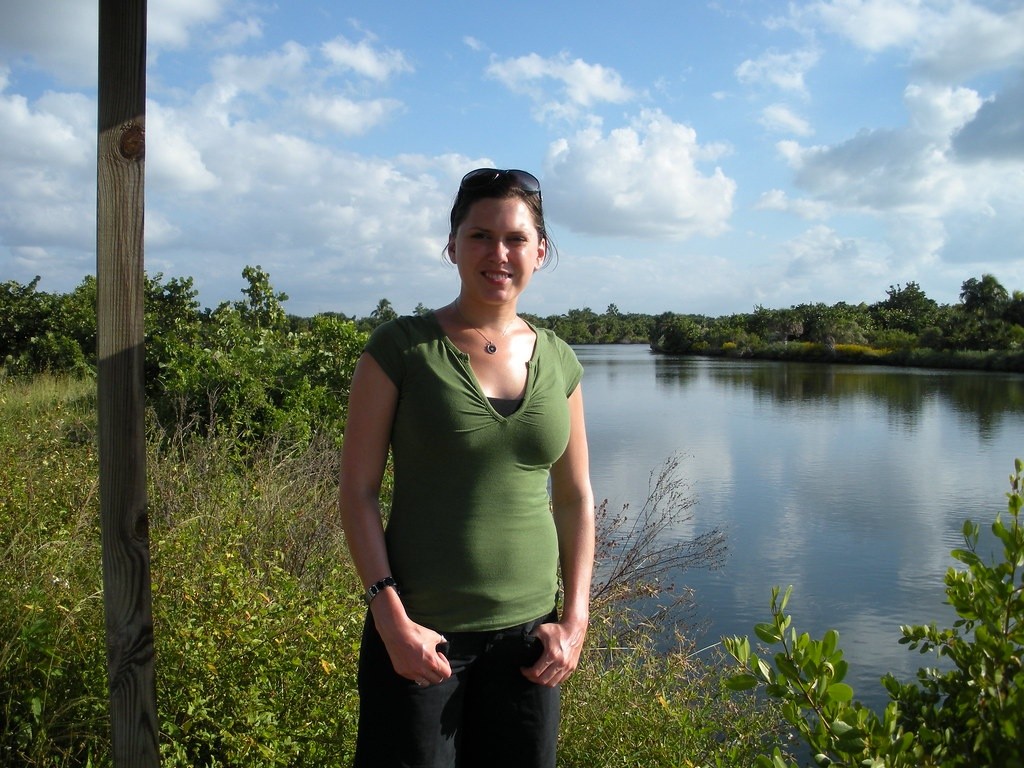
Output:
[362,576,401,605]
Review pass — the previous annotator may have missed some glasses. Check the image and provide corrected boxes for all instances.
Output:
[458,167,542,210]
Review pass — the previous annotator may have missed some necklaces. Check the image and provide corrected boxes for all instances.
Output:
[454,297,517,354]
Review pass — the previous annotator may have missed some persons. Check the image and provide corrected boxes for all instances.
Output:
[339,169,595,768]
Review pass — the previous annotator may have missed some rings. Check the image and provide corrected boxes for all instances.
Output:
[415,678,425,686]
[437,676,444,683]
